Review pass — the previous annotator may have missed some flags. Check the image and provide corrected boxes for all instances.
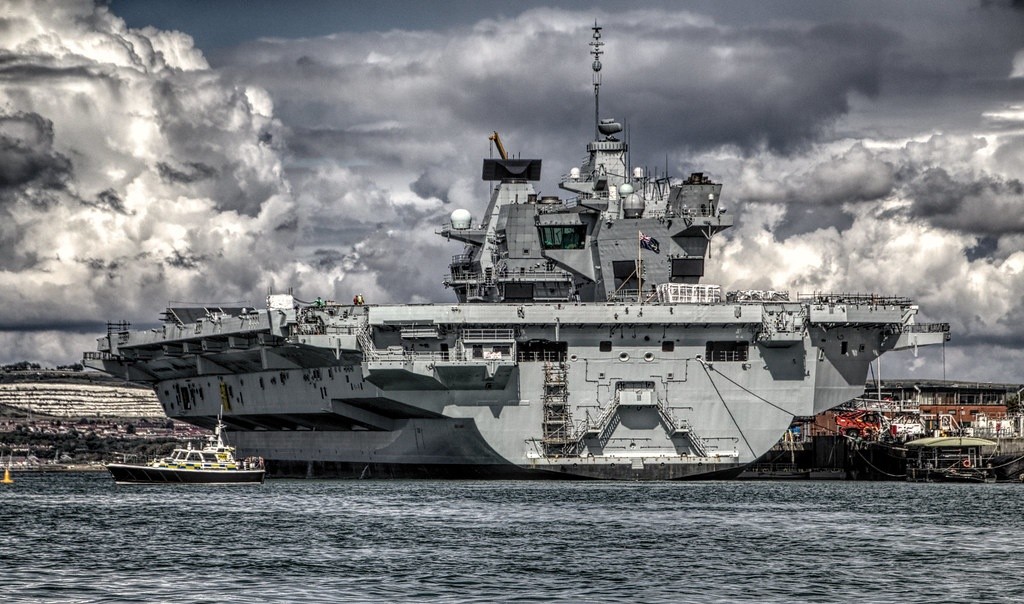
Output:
[639,231,660,254]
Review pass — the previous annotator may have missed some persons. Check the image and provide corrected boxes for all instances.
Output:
[312,296,324,304]
[240,307,247,315]
[356,292,364,304]
[352,294,358,304]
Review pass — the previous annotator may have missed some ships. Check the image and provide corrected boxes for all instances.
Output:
[81,18,919,481]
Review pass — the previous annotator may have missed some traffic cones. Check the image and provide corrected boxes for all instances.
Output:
[0,469,13,483]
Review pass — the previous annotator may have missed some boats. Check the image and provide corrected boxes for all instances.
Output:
[104,404,266,484]
